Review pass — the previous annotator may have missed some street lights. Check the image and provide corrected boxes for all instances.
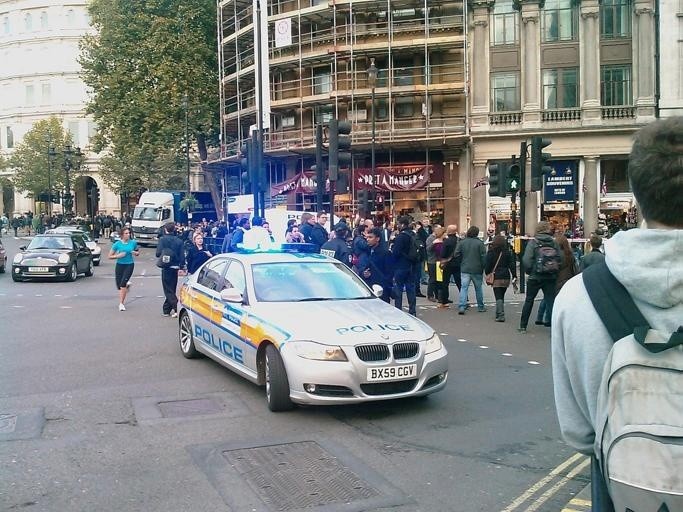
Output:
[48,143,87,219]
[363,57,380,216]
[180,89,205,199]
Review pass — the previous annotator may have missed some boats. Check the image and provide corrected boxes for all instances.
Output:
[529,135,552,192]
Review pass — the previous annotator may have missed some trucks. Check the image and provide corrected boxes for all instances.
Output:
[130,189,217,249]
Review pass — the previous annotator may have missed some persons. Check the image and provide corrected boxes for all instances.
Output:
[549,115,683,512]
[156,218,275,317]
[513,214,524,261]
[516,221,605,334]
[285,211,518,322]
[3,211,132,239]
[108,226,139,310]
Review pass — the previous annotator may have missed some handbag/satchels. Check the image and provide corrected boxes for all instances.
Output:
[156,248,175,268]
[485,271,496,285]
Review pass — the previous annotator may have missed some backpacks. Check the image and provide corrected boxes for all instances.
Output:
[580,260,683,512]
[399,230,425,264]
[531,237,563,276]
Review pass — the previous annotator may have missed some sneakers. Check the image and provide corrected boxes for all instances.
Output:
[118,303,126,311]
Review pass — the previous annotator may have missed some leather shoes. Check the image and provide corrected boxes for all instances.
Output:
[428,297,436,302]
[438,299,453,303]
[416,294,426,297]
[544,323,551,327]
[534,320,544,325]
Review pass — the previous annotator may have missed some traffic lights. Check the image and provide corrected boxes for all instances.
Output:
[485,160,505,198]
[327,118,352,181]
[505,158,520,194]
[240,138,252,184]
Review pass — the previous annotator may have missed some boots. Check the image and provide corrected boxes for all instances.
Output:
[494,298,505,323]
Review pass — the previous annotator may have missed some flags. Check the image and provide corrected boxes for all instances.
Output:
[601,175,607,198]
[473,175,490,191]
[582,182,588,193]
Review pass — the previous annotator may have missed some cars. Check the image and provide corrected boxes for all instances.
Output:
[9,233,94,283]
[0,210,102,274]
[173,239,451,414]
[108,222,134,244]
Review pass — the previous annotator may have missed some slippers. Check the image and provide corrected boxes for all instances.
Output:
[438,304,450,309]
